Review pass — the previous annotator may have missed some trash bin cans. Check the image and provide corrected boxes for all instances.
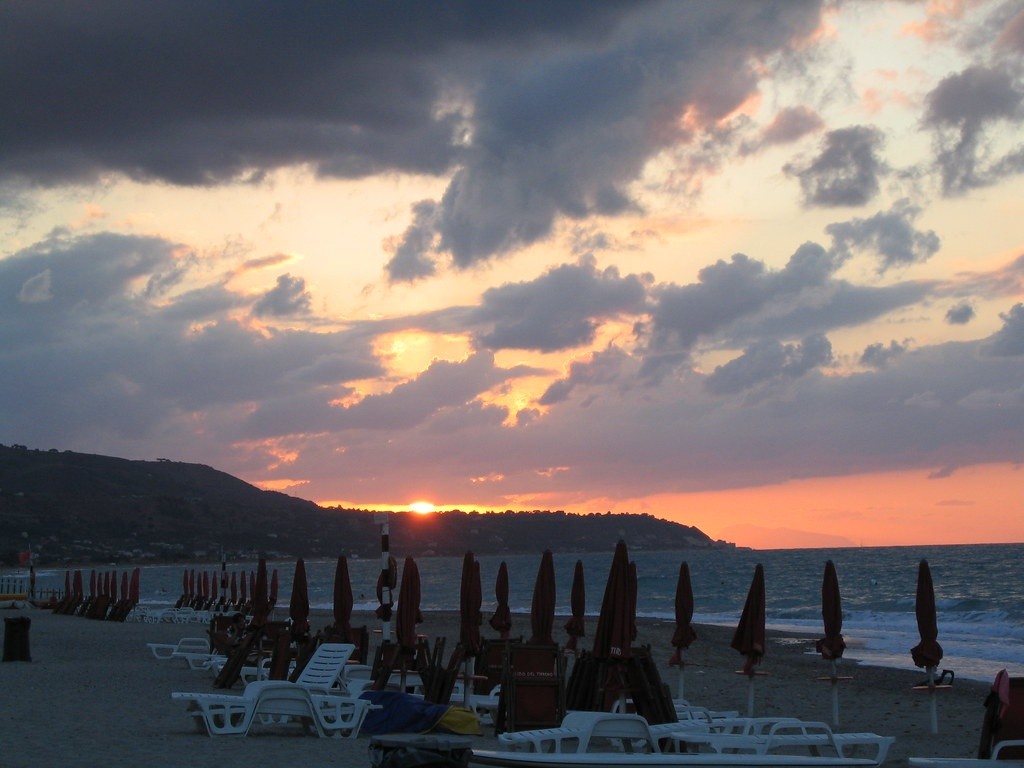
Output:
[992,677,1024,760]
[0,616,32,662]
[367,736,473,768]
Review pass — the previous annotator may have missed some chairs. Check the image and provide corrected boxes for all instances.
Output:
[170,642,499,744]
[146,637,225,673]
[124,607,240,624]
[500,699,1024,768]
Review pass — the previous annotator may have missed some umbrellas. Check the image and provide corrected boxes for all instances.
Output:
[334,558,354,630]
[395,555,423,692]
[458,552,482,708]
[183,568,217,603]
[591,539,639,714]
[65,571,70,597]
[232,558,278,623]
[289,557,309,625]
[670,561,698,701]
[98,567,140,604]
[374,556,398,632]
[816,558,847,723]
[527,548,558,650]
[564,560,587,649]
[89,570,96,597]
[910,559,943,734]
[731,565,767,716]
[73,571,83,597]
[29,564,36,599]
[489,562,511,638]
